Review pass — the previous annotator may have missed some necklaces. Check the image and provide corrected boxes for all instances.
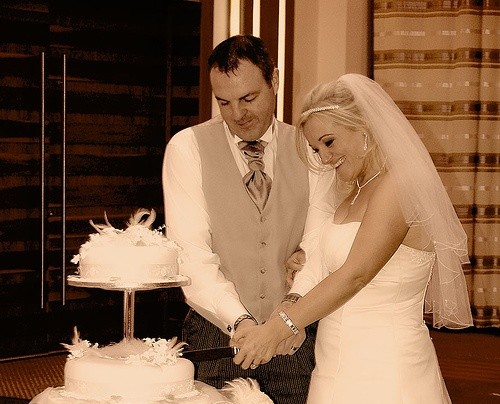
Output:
[350,154,388,208]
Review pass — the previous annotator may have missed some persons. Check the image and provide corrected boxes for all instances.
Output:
[162,35,316,404]
[229,73,475,404]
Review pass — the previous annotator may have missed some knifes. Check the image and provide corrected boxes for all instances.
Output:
[179,346,241,362]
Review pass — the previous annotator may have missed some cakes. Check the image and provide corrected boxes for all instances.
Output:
[31,328,230,402]
[72,209,179,283]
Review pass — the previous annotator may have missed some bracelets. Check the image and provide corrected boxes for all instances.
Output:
[279,311,299,335]
[233,314,253,328]
[280,295,301,303]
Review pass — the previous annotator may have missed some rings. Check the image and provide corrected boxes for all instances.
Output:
[250,363,258,369]
[291,345,297,350]
